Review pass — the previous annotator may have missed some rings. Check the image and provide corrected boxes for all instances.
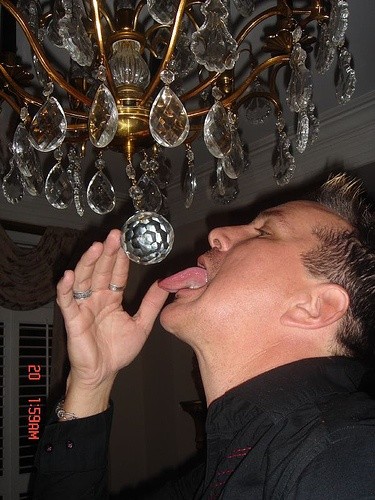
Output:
[72,285,93,298]
[108,282,127,291]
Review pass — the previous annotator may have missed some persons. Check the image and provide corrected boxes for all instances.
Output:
[24,168,375,500]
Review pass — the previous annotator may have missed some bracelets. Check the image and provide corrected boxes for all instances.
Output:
[53,399,79,420]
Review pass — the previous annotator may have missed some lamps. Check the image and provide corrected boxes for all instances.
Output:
[0,0,357,264]
